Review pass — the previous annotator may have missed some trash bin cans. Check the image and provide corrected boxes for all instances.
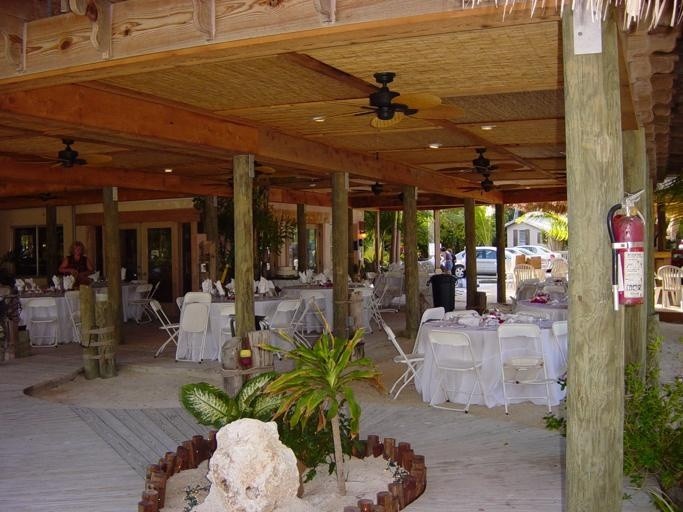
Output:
[430,274,457,313]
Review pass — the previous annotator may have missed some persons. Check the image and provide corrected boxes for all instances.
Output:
[447,248,456,276]
[440,247,454,275]
[58,241,94,277]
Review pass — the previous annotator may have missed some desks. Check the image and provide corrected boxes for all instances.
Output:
[417,316,569,406]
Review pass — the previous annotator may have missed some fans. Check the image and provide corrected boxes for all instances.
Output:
[352,180,400,195]
[201,176,234,188]
[387,192,430,205]
[12,137,113,182]
[316,72,464,127]
[438,145,523,173]
[456,174,520,192]
[196,160,275,176]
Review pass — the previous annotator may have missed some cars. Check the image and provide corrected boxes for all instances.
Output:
[477,248,537,260]
[512,245,561,270]
[452,245,546,278]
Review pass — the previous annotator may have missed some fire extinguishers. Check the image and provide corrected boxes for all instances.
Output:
[606,189,646,312]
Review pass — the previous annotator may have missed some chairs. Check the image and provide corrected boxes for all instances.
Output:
[380,321,429,397]
[499,324,551,414]
[653,265,683,309]
[149,299,180,359]
[176,266,440,364]
[430,330,488,412]
[1,277,162,349]
[514,266,569,316]
[551,320,568,370]
